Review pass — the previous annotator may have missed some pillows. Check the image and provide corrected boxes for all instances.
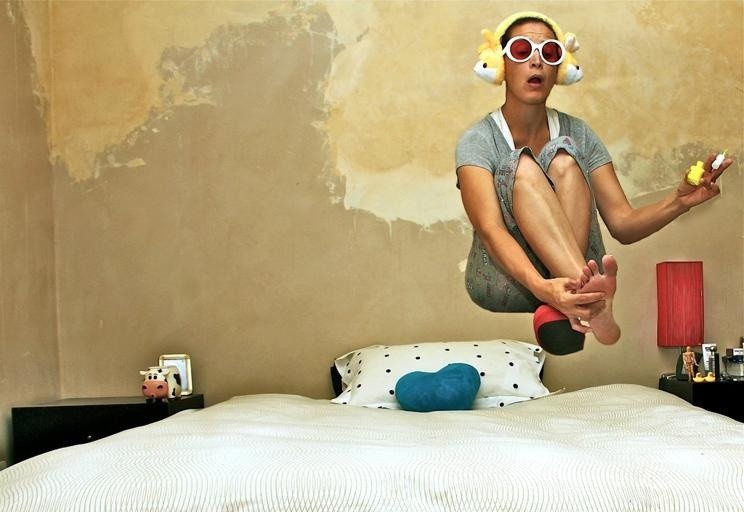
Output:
[329,339,552,413]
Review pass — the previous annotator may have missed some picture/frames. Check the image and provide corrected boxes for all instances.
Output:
[159,354,193,396]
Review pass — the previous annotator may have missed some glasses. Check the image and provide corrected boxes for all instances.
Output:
[503,35,567,68]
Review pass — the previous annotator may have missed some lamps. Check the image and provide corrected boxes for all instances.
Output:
[655,260,707,381]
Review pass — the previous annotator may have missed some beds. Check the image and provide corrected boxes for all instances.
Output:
[1,382,744,512]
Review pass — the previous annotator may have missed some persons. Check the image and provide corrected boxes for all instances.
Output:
[683,346,700,381]
[454,11,734,356]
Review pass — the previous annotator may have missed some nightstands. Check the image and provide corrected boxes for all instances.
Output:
[654,373,743,427]
[9,393,205,469]
